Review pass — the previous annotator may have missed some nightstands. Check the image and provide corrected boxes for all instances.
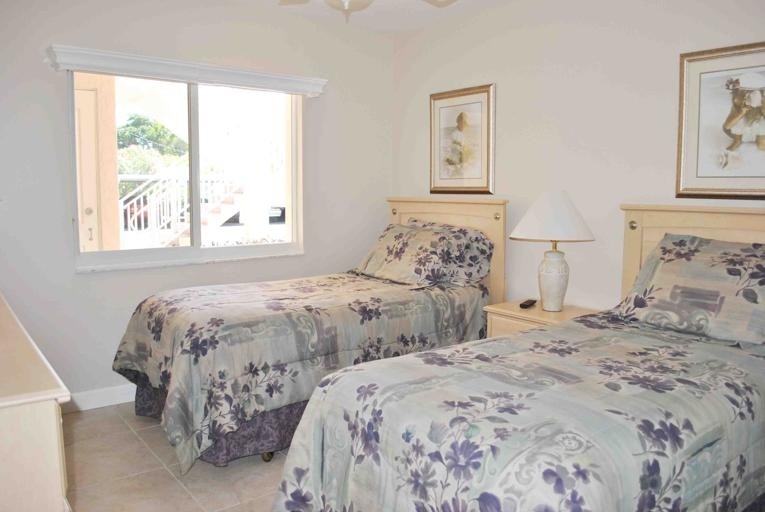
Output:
[484,300,600,338]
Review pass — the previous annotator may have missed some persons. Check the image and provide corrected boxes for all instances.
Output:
[723,73,765,152]
[445,111,470,178]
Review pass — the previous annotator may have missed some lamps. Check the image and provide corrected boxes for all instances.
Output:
[509,189,595,312]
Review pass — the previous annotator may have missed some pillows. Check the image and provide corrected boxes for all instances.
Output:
[612,234,765,343]
[354,222,465,290]
[407,217,492,287]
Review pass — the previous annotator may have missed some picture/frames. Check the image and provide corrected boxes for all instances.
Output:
[675,42,765,201]
[429,82,496,195]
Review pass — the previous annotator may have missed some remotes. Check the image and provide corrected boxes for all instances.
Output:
[519,299,537,308]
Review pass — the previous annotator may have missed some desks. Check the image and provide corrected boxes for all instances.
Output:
[0,294,73,512]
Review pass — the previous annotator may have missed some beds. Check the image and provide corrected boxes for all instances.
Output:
[113,198,507,476]
[268,206,765,512]
